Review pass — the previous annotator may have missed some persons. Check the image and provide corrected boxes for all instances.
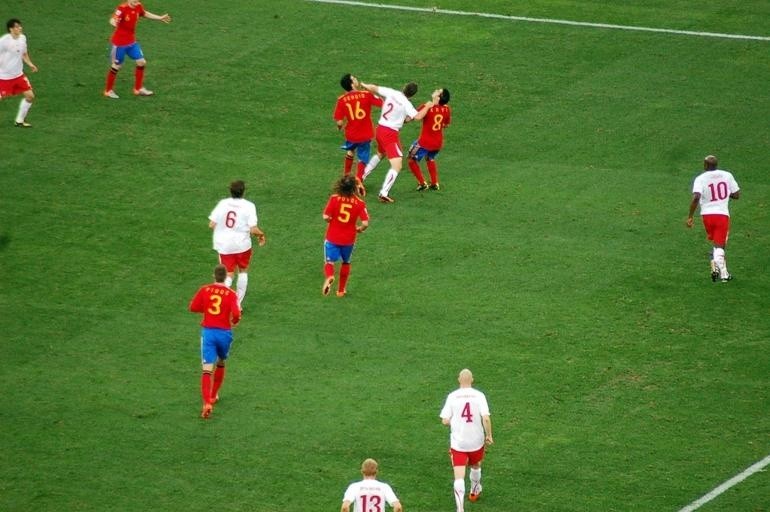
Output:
[333,73,385,198]
[208,180,266,313]
[322,174,370,299]
[102,0,172,99]
[686,155,741,283]
[0,18,38,128]
[404,88,451,192]
[188,265,241,417]
[359,81,434,204]
[439,368,494,512]
[339,458,403,512]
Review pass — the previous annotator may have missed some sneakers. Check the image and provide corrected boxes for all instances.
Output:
[428,183,439,190]
[469,484,482,502]
[710,259,720,282]
[201,404,212,418]
[322,275,335,296]
[211,393,218,403]
[103,88,119,99]
[14,121,31,128]
[355,177,366,197]
[416,183,428,191]
[378,194,393,202]
[133,87,153,96]
[336,290,346,296]
[721,274,733,282]
